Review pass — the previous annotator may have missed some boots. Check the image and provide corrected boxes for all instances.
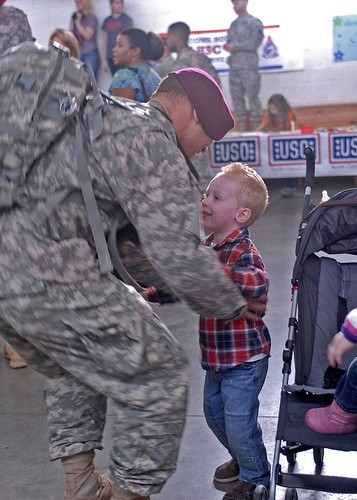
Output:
[62,449,114,500]
[306,399,357,434]
[111,482,150,500]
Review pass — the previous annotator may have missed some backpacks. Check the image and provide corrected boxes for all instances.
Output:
[0,41,135,210]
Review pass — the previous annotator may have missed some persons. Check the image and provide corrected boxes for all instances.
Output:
[0,66,235,500]
[161,22,221,90]
[153,37,175,79]
[138,162,272,500]
[306,308,356,435]
[49,28,81,63]
[68,0,100,84]
[222,0,265,132]
[106,27,161,104]
[255,93,296,134]
[100,0,134,77]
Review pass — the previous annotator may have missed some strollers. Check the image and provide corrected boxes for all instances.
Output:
[270,147,357,500]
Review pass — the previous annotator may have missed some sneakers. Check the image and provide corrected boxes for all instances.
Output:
[223,480,270,500]
[214,459,239,482]
[4,344,27,368]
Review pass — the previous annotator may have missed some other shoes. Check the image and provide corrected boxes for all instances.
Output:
[238,122,260,132]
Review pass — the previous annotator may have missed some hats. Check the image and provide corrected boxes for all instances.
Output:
[168,67,234,141]
